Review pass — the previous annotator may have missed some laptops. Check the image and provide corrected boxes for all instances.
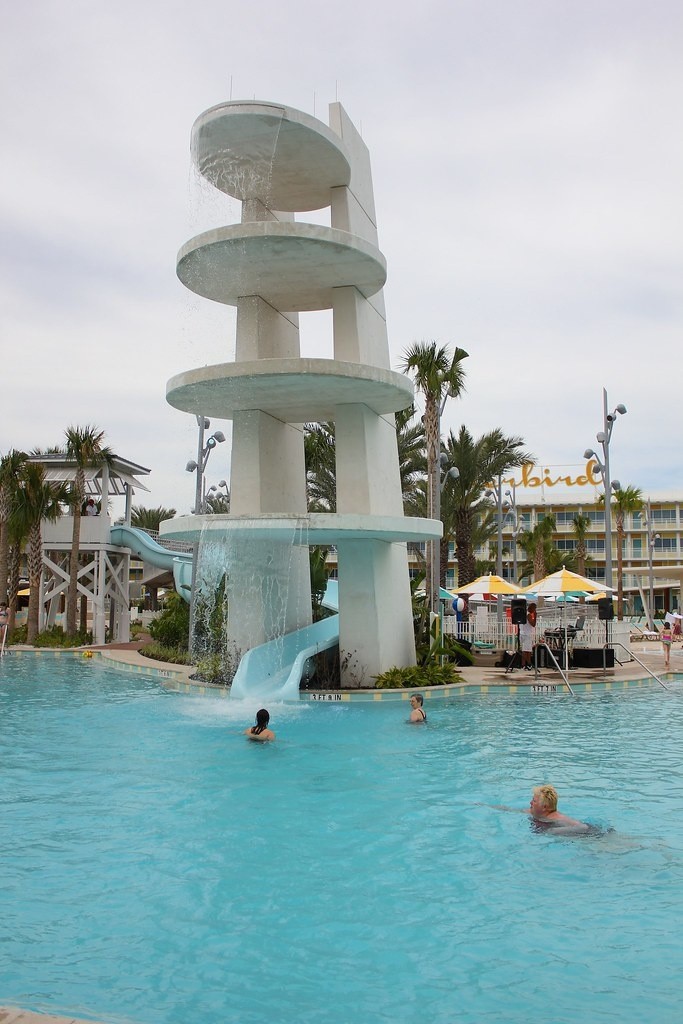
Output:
[570,616,585,629]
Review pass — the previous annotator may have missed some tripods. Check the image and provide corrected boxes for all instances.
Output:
[504,624,540,674]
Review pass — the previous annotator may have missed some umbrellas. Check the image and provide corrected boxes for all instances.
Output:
[511,592,624,603]
[412,587,457,599]
[518,565,617,667]
[468,593,505,600]
[452,571,521,644]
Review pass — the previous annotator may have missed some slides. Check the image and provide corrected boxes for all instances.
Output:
[109,524,340,702]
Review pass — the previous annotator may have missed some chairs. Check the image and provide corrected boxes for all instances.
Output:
[633,623,661,641]
[630,616,648,626]
[652,618,665,633]
[629,623,652,641]
[661,619,683,641]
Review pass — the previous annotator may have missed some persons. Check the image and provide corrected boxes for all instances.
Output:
[673,618,681,642]
[661,622,672,664]
[519,603,536,670]
[410,694,426,722]
[85,499,97,515]
[0,602,11,650]
[246,709,275,740]
[529,785,581,824]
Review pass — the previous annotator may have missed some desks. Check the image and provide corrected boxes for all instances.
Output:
[470,647,510,667]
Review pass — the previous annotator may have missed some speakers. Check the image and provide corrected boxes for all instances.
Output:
[598,597,614,620]
[548,649,570,668]
[510,599,527,624]
